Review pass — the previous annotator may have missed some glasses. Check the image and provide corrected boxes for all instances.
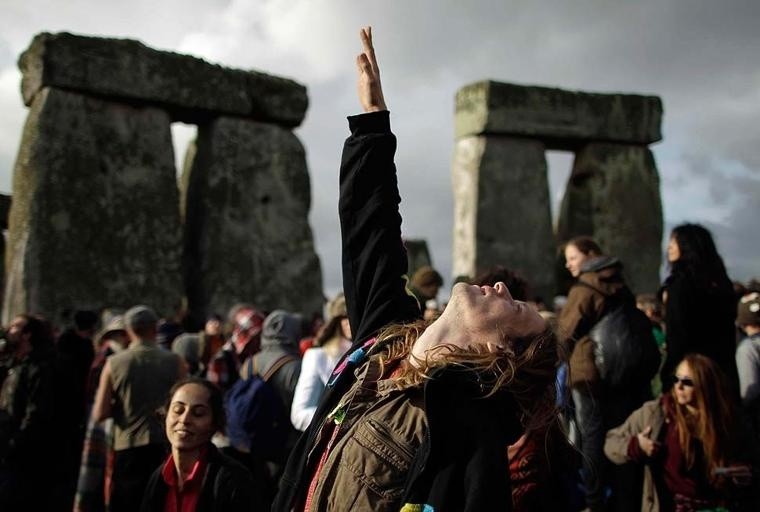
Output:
[671,375,694,386]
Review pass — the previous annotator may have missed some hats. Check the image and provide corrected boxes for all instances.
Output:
[734,291,760,324]
[259,309,304,349]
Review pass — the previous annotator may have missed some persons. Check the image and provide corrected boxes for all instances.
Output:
[1,290,352,512]
[412,267,444,316]
[267,25,602,511]
[483,222,760,512]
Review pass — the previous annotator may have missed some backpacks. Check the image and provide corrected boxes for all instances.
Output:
[220,353,301,453]
[572,281,661,386]
